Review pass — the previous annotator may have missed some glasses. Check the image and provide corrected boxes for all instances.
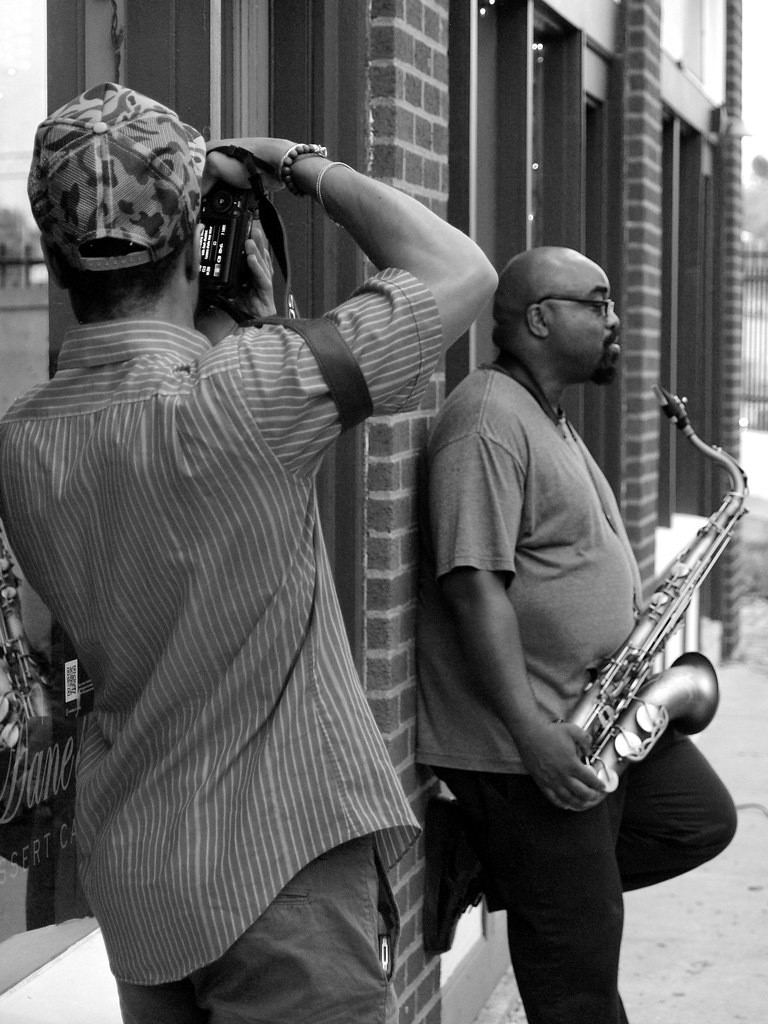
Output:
[536,294,615,318]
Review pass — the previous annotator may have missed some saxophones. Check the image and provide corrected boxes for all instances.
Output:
[541,380,751,814]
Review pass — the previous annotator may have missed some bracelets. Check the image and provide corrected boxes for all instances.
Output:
[279,142,328,198]
[316,162,357,229]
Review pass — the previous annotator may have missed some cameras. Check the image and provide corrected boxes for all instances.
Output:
[194,180,264,310]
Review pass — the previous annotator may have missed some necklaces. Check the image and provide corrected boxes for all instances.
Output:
[558,419,617,535]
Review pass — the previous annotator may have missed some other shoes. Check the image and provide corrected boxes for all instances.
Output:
[424,797,477,955]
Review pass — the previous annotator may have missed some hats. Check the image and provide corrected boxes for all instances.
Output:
[27,82,208,271]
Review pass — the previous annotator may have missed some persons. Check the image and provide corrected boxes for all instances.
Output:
[413,246,736,1024]
[0,82,500,1022]
